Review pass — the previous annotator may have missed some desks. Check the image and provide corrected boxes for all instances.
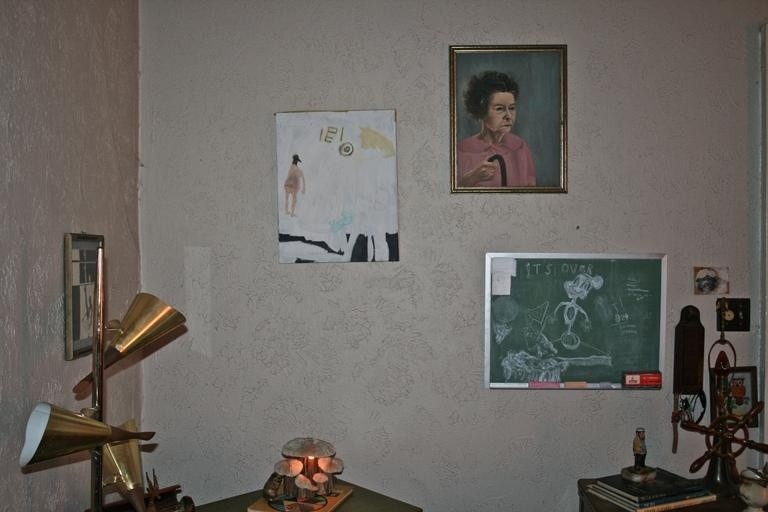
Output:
[577,478,748,512]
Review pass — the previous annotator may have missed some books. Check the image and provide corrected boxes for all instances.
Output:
[582,462,717,512]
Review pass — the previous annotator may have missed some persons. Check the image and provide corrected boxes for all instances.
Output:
[632,426,649,473]
[455,70,545,192]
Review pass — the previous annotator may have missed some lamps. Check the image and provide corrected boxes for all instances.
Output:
[18,245,189,512]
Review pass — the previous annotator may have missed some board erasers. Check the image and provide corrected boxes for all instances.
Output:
[622,370,662,390]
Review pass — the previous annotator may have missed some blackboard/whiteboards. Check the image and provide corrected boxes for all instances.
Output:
[483,252,667,391]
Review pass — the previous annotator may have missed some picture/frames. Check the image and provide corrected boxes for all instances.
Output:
[63,231,108,362]
[709,365,759,429]
[448,43,569,195]
[716,298,750,332]
